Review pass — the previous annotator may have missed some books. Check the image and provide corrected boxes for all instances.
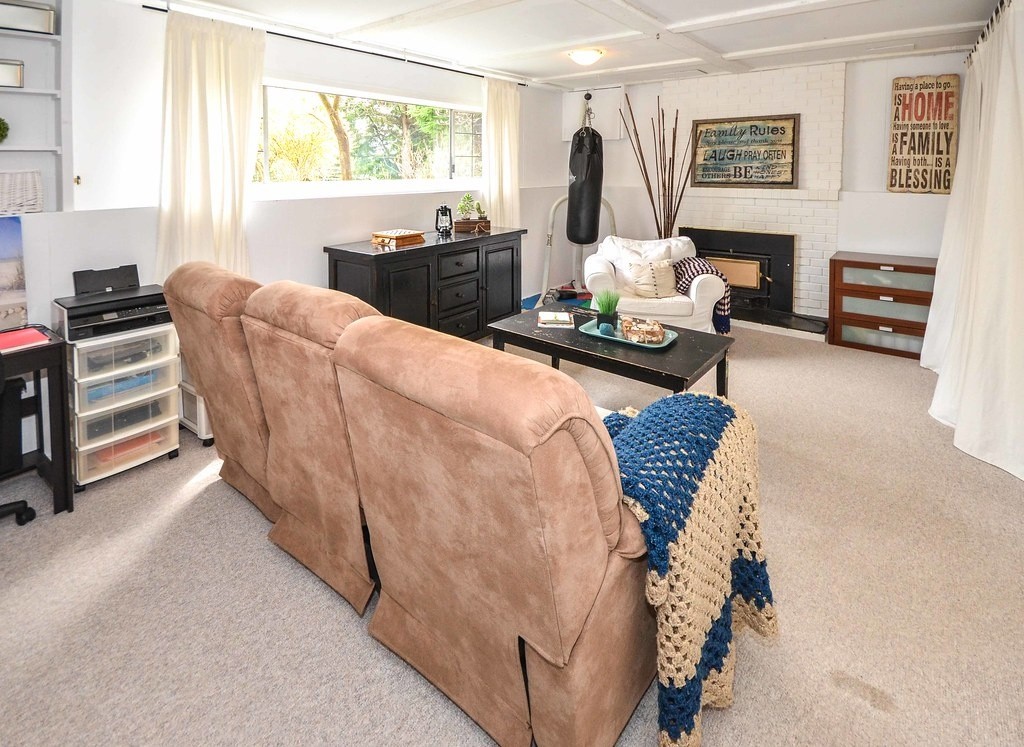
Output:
[538,312,575,330]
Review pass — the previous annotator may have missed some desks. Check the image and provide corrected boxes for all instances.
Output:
[0,324,68,514]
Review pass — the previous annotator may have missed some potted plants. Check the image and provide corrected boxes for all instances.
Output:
[457,193,475,221]
[476,202,488,220]
[595,291,621,332]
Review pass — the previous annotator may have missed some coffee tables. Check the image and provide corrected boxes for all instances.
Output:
[488,302,735,402]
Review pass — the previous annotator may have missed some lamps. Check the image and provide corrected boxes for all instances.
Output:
[568,48,603,66]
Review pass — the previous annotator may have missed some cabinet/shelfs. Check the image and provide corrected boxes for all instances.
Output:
[64,323,181,493]
[829,250,939,361]
[0,0,75,301]
[324,226,528,342]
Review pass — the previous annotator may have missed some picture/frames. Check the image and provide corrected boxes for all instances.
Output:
[690,113,799,188]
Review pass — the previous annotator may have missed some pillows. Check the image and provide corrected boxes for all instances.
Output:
[624,259,682,299]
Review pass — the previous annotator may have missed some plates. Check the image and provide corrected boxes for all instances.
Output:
[579,318,678,348]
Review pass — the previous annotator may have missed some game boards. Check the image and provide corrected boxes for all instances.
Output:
[371,228,426,239]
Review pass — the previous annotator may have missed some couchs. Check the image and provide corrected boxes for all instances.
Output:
[585,236,725,336]
[162,259,778,747]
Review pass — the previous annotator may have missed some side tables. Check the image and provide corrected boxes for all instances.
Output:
[181,355,215,448]
